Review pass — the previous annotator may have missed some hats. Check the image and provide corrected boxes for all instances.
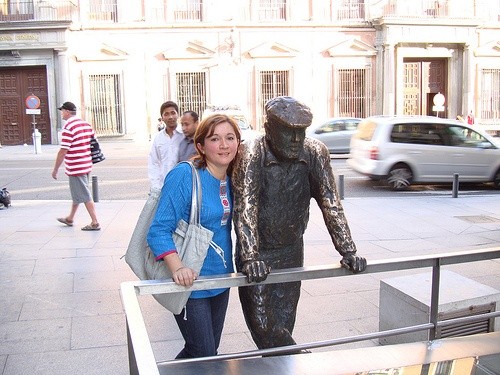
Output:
[57,101,76,111]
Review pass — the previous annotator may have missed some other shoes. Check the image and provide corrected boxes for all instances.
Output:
[81,224,101,230]
[56,216,73,226]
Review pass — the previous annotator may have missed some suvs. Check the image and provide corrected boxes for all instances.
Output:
[344,114,500,192]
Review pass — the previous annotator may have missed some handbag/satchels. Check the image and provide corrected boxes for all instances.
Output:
[125,161,214,315]
[90,139,105,164]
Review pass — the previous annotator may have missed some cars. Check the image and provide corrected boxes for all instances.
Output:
[311,116,366,156]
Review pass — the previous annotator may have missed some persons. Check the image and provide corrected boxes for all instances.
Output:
[157,117,166,132]
[52,102,102,231]
[232,95,369,357]
[146,114,242,360]
[177,111,199,162]
[457,112,475,139]
[148,101,185,192]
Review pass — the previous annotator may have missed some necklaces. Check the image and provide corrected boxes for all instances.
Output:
[207,167,226,180]
